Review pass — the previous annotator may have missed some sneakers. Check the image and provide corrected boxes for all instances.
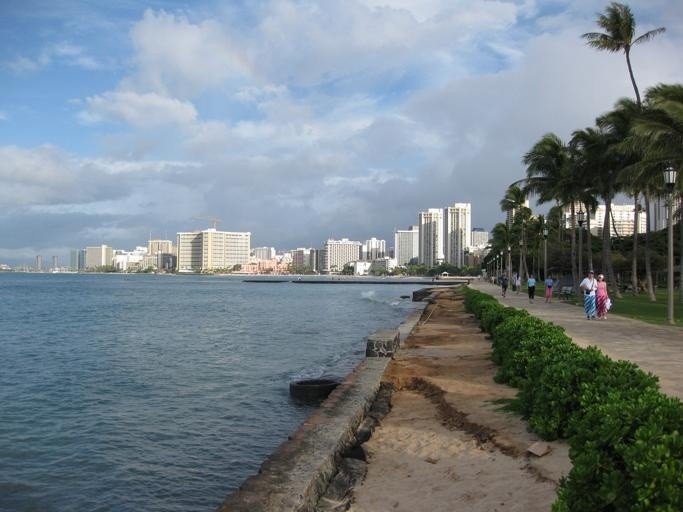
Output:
[586,316,606,320]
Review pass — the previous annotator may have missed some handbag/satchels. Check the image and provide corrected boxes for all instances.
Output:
[586,290,590,294]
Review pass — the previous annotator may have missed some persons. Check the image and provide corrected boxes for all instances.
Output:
[499,270,608,320]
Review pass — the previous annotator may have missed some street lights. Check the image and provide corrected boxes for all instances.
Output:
[519,239,524,283]
[487,246,511,289]
[577,209,584,307]
[543,226,549,285]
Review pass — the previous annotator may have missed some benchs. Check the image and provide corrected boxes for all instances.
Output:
[557,286,574,300]
